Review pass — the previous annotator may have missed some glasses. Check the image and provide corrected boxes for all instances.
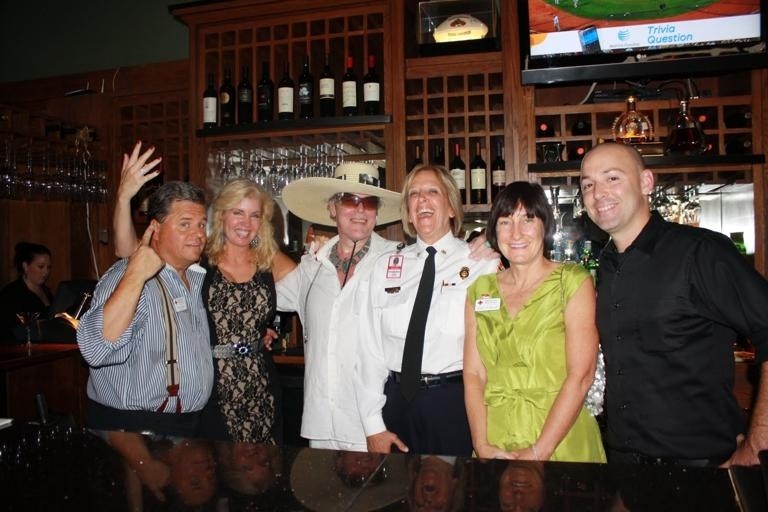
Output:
[332,192,379,211]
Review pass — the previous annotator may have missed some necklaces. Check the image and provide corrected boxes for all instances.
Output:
[328,237,371,275]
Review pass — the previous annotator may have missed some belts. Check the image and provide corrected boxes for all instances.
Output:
[209,341,259,358]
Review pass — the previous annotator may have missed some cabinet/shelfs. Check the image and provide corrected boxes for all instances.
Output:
[169,3,390,133]
[393,1,515,235]
[106,60,190,247]
[518,63,768,178]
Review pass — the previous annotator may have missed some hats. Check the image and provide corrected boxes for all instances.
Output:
[281,162,404,226]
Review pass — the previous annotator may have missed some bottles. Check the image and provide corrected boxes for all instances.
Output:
[431,144,443,166]
[612,96,652,145]
[571,146,584,159]
[278,63,294,117]
[203,72,218,127]
[555,238,598,270]
[238,64,253,124]
[410,145,421,171]
[343,57,358,116]
[297,55,312,119]
[666,96,706,157]
[257,62,273,122]
[449,144,466,205]
[571,122,590,135]
[319,53,336,117]
[364,55,382,116]
[538,121,556,137]
[220,69,235,126]
[471,143,488,203]
[491,143,505,203]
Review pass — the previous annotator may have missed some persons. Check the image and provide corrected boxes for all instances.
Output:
[579,141,768,469]
[274,161,503,453]
[122,435,220,512]
[461,180,609,463]
[1,241,55,343]
[405,453,465,512]
[492,459,548,512]
[100,430,283,501]
[355,164,503,462]
[76,179,217,430]
[112,139,298,445]
[289,447,410,512]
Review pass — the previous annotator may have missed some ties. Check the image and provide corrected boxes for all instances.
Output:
[400,245,438,401]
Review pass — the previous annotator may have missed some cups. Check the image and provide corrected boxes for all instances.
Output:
[16,311,40,362]
